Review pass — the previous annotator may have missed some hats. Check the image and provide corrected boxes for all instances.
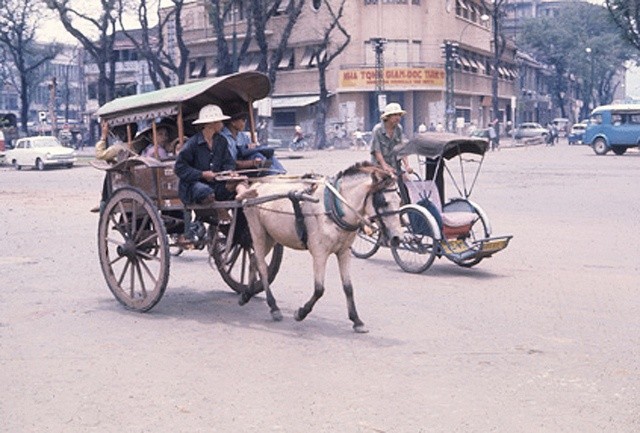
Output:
[134,116,177,139]
[222,103,248,125]
[191,104,232,125]
[171,136,190,155]
[380,103,406,120]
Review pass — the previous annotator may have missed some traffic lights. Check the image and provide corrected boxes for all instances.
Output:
[441,42,459,60]
[372,38,386,53]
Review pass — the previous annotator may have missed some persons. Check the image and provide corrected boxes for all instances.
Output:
[418,120,456,139]
[468,123,498,152]
[548,121,571,144]
[72,131,86,151]
[175,104,245,225]
[220,112,284,181]
[165,133,186,165]
[89,120,138,213]
[366,103,407,245]
[141,123,170,170]
[290,126,304,149]
[330,123,364,149]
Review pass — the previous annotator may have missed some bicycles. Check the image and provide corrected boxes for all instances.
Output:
[289,139,308,152]
[354,138,366,151]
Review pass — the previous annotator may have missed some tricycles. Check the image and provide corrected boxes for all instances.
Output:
[349,133,514,278]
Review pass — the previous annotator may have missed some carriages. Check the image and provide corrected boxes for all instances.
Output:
[90,72,396,332]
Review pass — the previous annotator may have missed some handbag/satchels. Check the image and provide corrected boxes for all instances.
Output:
[235,143,274,177]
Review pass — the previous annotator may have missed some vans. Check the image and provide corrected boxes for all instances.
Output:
[584,105,640,155]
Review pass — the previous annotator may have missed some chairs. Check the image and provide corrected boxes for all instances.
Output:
[405,177,477,239]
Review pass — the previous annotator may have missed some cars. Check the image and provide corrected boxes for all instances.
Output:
[569,123,586,145]
[517,122,551,138]
[7,136,77,170]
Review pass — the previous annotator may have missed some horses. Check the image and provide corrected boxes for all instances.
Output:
[243,161,405,333]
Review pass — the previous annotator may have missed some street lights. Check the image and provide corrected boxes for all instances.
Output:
[459,13,489,118]
[511,97,516,149]
[570,48,591,126]
